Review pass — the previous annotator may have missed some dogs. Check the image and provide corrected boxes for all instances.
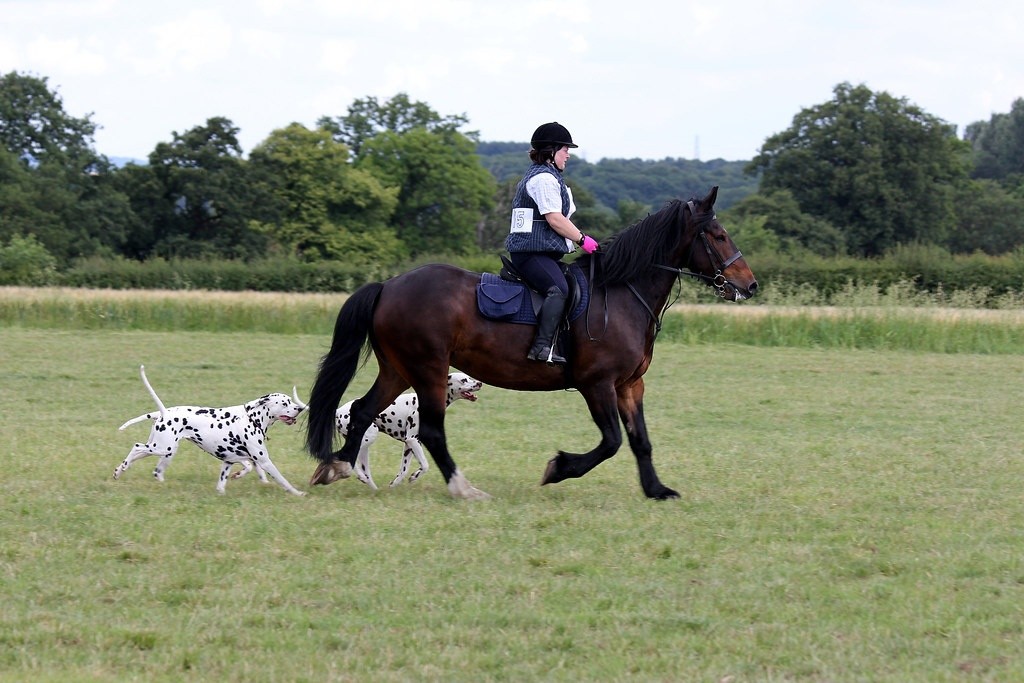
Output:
[113,364,309,498]
[292,373,483,490]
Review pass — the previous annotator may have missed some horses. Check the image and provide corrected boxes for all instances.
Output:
[299,187,759,502]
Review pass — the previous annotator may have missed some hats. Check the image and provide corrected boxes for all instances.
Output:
[533,122,578,149]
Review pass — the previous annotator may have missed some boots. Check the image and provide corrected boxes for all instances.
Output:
[526,286,567,366]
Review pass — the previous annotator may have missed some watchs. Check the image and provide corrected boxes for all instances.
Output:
[576,231,585,248]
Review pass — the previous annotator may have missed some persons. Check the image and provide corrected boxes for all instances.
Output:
[504,121,601,364]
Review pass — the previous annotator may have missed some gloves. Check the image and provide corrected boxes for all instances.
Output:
[578,234,597,253]
